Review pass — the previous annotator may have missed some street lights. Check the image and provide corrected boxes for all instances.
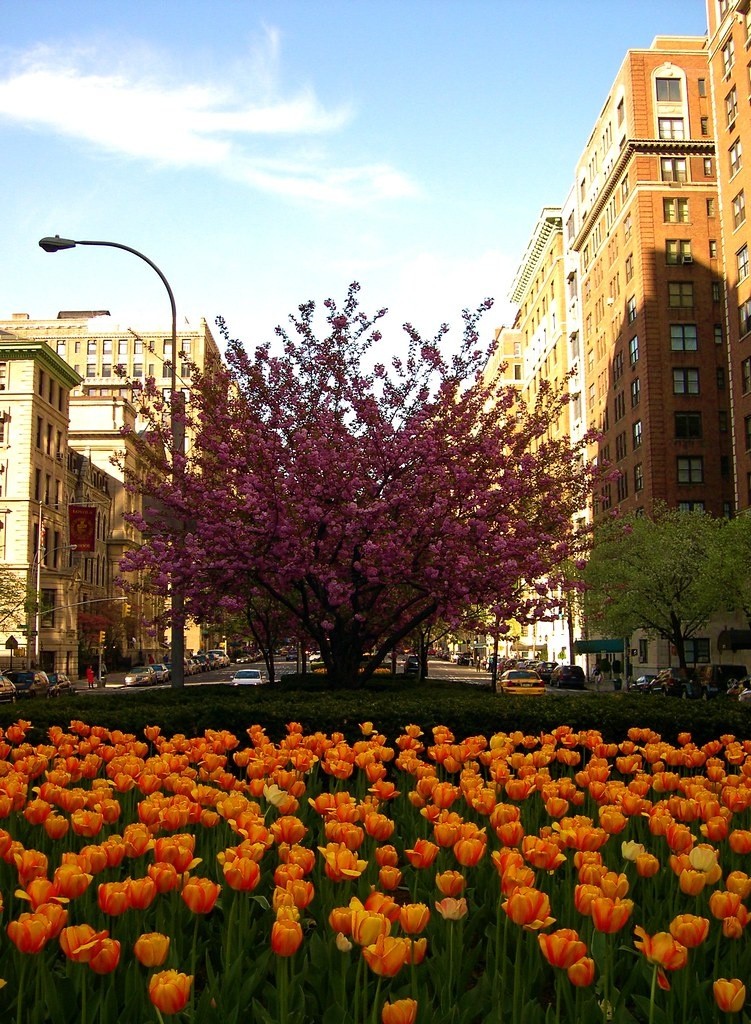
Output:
[38,234,187,689]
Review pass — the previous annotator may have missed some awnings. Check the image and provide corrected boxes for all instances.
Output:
[574,639,624,653]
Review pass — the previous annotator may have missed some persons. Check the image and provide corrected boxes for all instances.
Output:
[475,655,528,675]
[98,661,108,677]
[592,664,602,692]
[189,649,204,656]
[149,654,169,664]
[86,665,95,689]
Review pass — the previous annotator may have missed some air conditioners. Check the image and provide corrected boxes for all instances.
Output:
[56,452,64,460]
[72,469,79,475]
[607,298,613,306]
[681,257,692,264]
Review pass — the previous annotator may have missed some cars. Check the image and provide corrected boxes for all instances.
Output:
[0,676,17,704]
[126,667,158,687]
[428,649,538,673]
[496,669,547,696]
[145,663,168,681]
[47,673,74,698]
[631,667,751,703]
[165,646,322,678]
[230,669,267,686]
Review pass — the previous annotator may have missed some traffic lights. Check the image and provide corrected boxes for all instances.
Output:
[100,631,105,642]
[27,544,77,664]
[122,602,132,617]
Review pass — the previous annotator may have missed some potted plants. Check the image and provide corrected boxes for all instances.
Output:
[598,658,611,680]
[612,660,623,679]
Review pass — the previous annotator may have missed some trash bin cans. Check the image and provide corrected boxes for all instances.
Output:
[100,677,106,688]
[613,678,622,690]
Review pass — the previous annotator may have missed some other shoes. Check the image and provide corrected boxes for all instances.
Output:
[92,687,95,689]
[89,687,91,689]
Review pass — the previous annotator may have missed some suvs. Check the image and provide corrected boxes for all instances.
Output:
[1,668,51,700]
[404,654,429,675]
[680,665,747,700]
[534,662,558,680]
[549,666,585,689]
